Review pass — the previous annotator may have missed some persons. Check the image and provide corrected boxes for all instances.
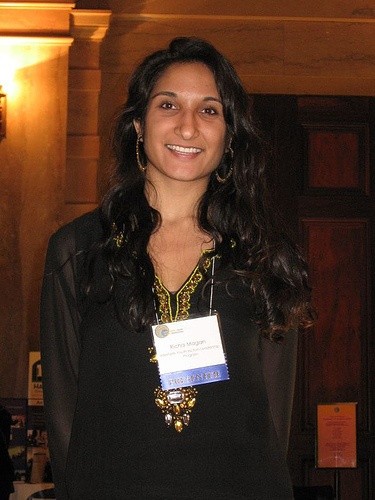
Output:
[39,35,319,499]
